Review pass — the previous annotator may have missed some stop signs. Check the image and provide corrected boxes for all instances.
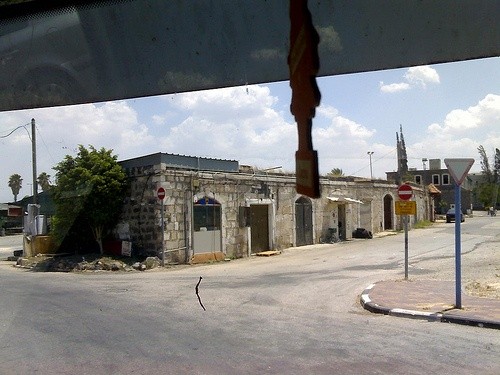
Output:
[157,187,165,199]
[397,184,414,201]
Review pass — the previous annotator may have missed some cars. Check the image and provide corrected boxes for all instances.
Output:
[446,207,465,222]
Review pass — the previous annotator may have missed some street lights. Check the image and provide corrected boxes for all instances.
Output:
[367,152,374,178]
